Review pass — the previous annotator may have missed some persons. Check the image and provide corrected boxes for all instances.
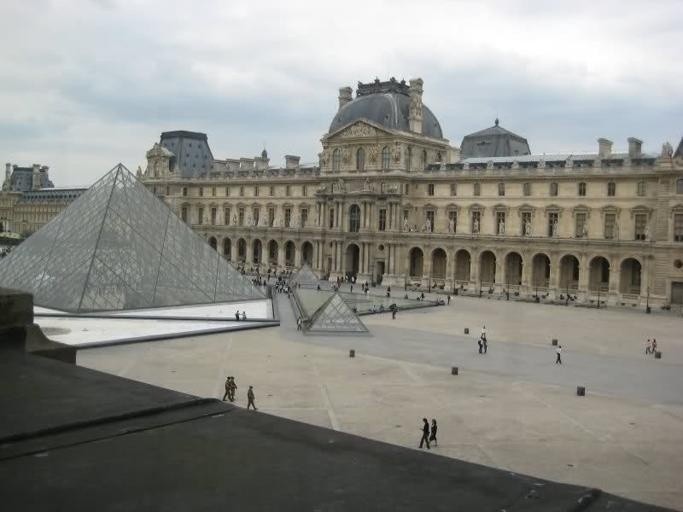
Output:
[222,376,233,402]
[651,338,656,353]
[428,418,438,448]
[234,310,240,320]
[554,345,561,364]
[644,338,651,354]
[295,315,302,331]
[235,267,356,294]
[229,375,236,399]
[477,325,487,354]
[241,311,246,320]
[246,385,258,412]
[417,417,430,449]
